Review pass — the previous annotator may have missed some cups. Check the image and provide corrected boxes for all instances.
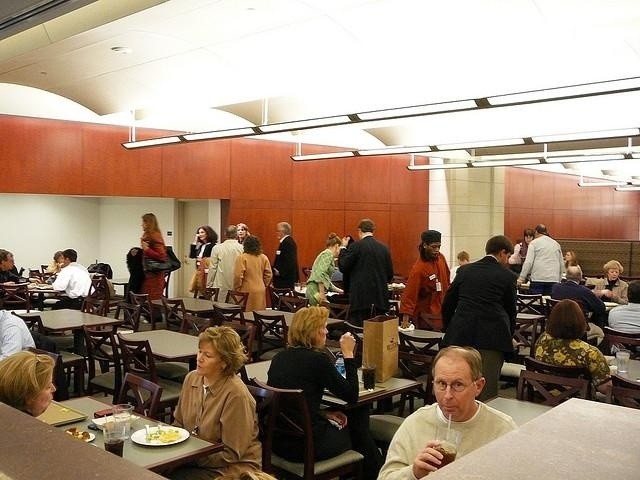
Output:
[614,349,631,375]
[111,402,132,434]
[433,427,461,468]
[360,360,377,389]
[102,424,126,458]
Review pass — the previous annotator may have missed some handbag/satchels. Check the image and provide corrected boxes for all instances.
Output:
[142,246,180,273]
[362,315,399,383]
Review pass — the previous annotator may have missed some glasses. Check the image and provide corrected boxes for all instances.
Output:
[432,381,468,392]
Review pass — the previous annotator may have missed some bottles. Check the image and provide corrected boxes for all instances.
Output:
[334,353,347,384]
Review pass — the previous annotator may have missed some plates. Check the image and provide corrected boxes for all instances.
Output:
[131,424,190,450]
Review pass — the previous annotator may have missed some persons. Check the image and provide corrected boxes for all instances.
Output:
[376,345,517,480]
[534,300,614,407]
[0,310,36,362]
[170,326,263,480]
[260,307,385,480]
[442,236,516,402]
[305,217,470,330]
[130,214,169,323]
[188,222,300,312]
[0,248,95,310]
[509,224,640,355]
[0,351,57,417]
